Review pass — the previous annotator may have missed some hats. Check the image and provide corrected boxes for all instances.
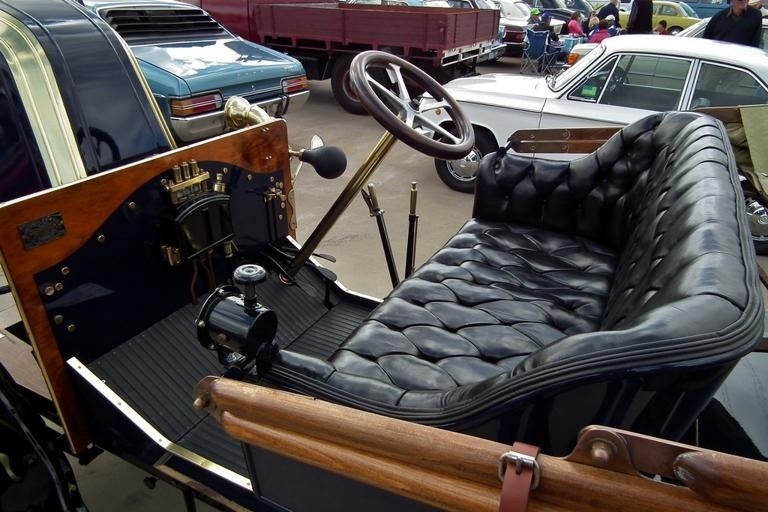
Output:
[529,8,541,15]
[604,14,615,20]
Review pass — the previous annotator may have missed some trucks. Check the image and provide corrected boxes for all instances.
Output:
[178,0,507,117]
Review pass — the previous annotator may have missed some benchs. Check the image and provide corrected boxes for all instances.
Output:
[272,109,768,456]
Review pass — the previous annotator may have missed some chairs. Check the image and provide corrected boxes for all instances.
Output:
[691,96,711,110]
[520,29,560,75]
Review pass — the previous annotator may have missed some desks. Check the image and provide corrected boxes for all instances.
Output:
[550,34,588,53]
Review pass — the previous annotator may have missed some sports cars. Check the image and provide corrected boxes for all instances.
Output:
[0,0,768,512]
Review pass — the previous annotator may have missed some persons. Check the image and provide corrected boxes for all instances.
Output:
[564,11,626,43]
[596,1,621,28]
[530,12,560,75]
[702,0,761,48]
[748,0,767,19]
[624,1,652,33]
[525,8,541,24]
[654,20,671,35]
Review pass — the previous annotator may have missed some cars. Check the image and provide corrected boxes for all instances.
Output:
[566,16,768,98]
[74,0,310,143]
[416,33,768,193]
[340,0,731,61]
